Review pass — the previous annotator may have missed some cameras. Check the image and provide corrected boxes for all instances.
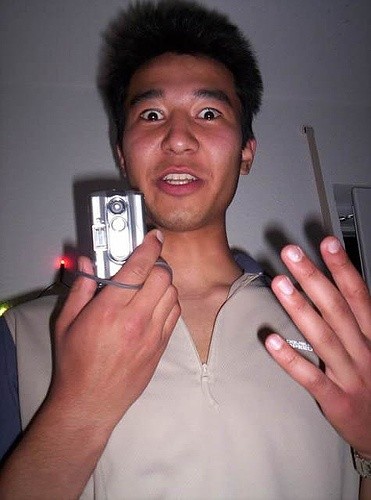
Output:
[85,191,145,288]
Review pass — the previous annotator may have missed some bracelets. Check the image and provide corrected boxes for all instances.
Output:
[352,448,371,483]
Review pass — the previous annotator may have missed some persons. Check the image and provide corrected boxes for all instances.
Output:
[0,0,371,500]
